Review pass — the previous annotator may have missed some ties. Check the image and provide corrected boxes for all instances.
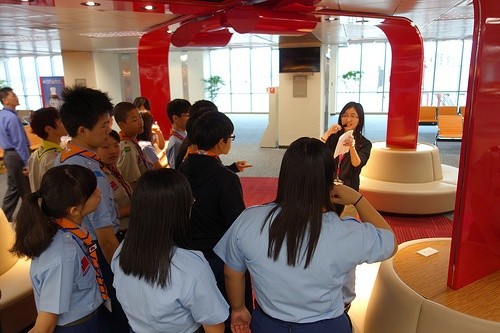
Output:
[119,130,153,172]
[60,140,104,170]
[100,161,131,199]
[52,216,107,300]
[37,141,62,160]
[170,129,185,142]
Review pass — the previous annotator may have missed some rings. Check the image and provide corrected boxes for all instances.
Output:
[335,128,337,130]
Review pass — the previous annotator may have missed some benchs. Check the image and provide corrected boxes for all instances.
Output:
[418,105,465,146]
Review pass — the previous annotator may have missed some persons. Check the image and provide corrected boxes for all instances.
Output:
[0,86,31,223]
[54,86,130,332]
[27,106,68,208]
[136,110,169,171]
[7,164,112,333]
[96,130,133,234]
[132,96,150,112]
[176,100,253,174]
[320,102,372,217]
[113,102,148,185]
[213,137,398,333]
[166,99,191,170]
[111,168,230,333]
[179,111,251,333]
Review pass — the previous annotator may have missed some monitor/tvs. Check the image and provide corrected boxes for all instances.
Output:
[279,46,321,73]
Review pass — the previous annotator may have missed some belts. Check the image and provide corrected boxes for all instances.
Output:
[57,303,104,327]
[5,148,15,151]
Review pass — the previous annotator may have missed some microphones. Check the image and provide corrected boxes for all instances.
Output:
[341,123,348,128]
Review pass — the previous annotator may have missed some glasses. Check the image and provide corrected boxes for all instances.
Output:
[341,113,359,121]
[227,134,235,141]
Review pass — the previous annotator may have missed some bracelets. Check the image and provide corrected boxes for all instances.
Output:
[354,194,364,206]
[321,137,327,141]
